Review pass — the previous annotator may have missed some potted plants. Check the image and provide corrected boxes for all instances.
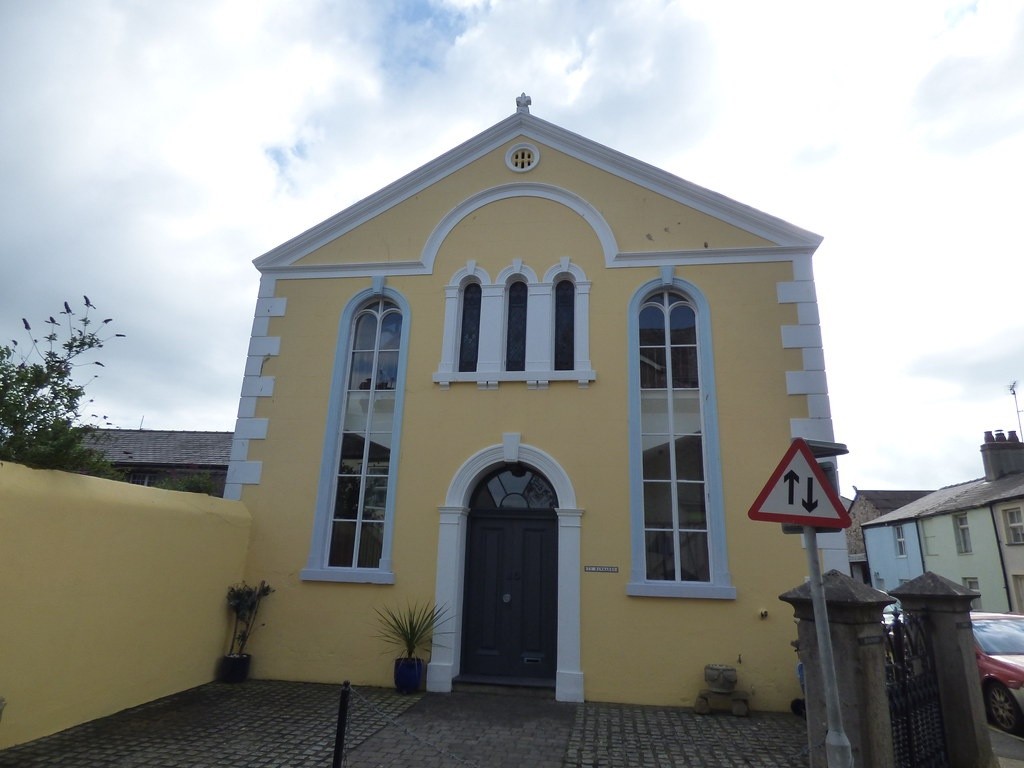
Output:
[367,600,459,694]
[218,580,276,683]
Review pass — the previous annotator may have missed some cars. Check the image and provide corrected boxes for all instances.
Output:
[886,610,1024,732]
